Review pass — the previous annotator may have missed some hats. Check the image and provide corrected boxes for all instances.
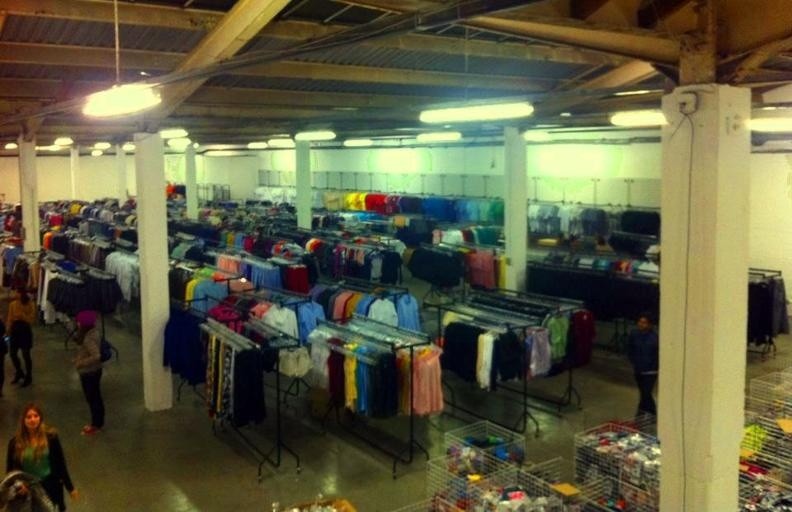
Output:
[73,311,96,325]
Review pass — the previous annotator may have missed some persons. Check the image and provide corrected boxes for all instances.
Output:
[75,311,105,436]
[6,286,35,386]
[185,238,205,261]
[627,316,660,416]
[251,226,265,257]
[7,403,78,501]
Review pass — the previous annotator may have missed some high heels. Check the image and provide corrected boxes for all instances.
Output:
[12,370,32,387]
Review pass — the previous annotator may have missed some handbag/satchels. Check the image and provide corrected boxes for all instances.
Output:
[100,339,111,361]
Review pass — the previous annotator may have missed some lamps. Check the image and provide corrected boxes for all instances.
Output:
[80,2,161,118]
[419,27,534,124]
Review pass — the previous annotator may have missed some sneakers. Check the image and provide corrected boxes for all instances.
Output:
[81,423,103,435]
